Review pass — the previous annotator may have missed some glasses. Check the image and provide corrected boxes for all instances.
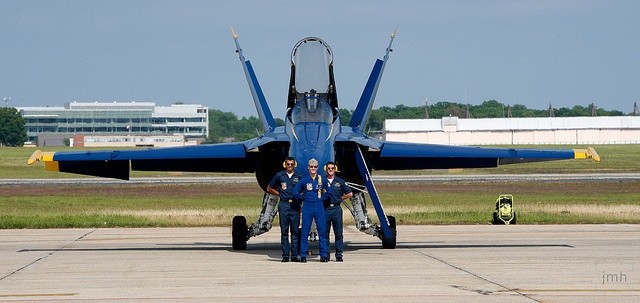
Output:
[310,165,318,168]
[286,163,295,166]
[328,167,334,170]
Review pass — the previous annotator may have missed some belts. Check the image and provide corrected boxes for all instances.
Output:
[329,203,339,207]
[280,199,295,203]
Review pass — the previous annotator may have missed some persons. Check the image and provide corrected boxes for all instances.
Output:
[321,161,353,262]
[267,157,303,262]
[293,158,332,262]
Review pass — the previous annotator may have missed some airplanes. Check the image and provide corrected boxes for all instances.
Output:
[28,25,600,248]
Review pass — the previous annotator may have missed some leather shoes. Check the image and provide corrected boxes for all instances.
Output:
[320,257,330,261]
[323,257,328,262]
[291,257,300,262]
[336,258,343,261]
[282,257,289,262]
[301,257,306,262]
